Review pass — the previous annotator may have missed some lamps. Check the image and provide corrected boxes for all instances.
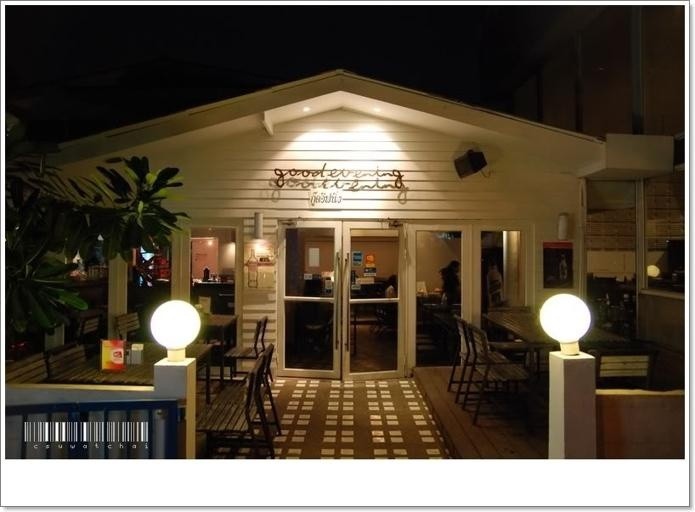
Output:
[252,211,264,240]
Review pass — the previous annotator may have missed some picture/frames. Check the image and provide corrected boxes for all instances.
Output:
[540,240,577,292]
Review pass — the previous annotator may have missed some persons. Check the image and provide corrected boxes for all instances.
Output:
[444,260,460,305]
[384,274,397,298]
[438,268,448,305]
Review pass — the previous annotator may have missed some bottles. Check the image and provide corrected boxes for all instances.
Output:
[559,255,568,280]
[248,248,257,289]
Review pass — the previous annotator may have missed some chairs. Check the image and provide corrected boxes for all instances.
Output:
[4,268,684,459]
